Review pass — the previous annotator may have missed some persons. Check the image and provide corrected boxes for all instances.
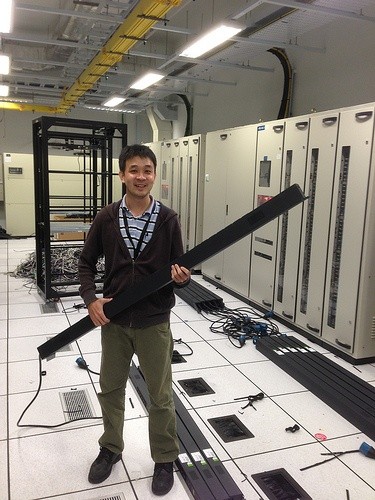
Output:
[77,145,191,495]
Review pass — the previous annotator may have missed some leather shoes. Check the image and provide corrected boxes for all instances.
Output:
[87,448,122,483]
[152,462,174,496]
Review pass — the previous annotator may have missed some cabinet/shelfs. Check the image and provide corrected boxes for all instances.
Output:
[199,122,260,295]
[3,153,122,237]
[140,141,163,199]
